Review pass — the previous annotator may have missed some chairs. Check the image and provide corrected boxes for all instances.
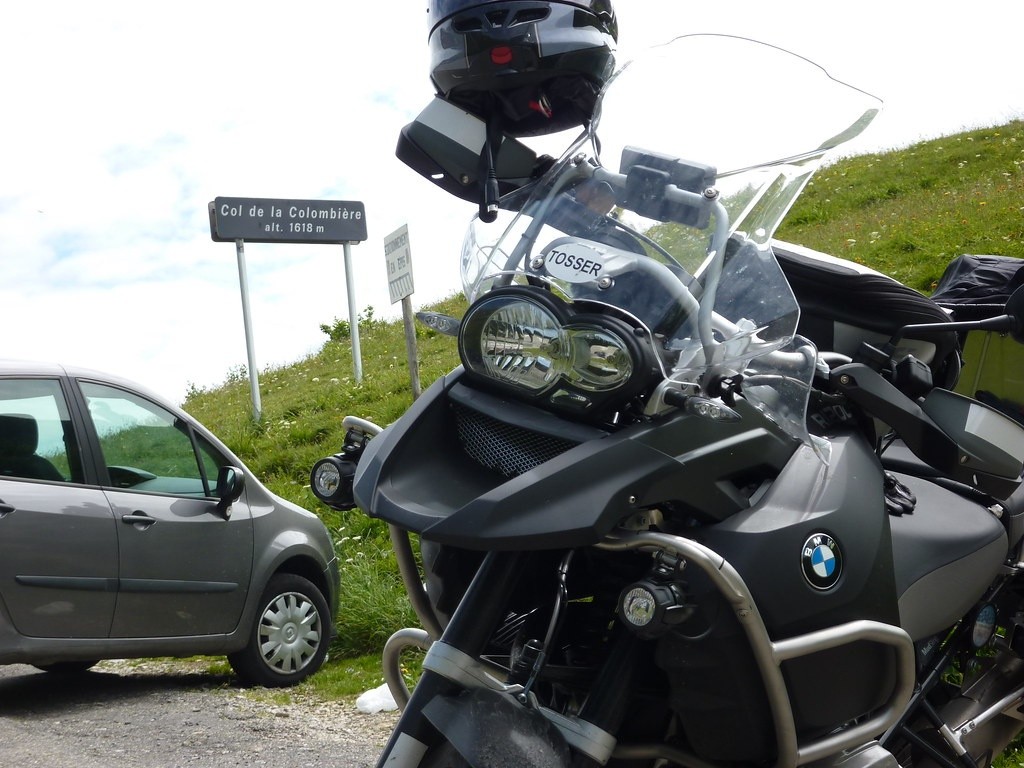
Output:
[0,413,68,482]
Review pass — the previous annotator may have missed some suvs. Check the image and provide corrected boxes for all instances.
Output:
[0,366,345,687]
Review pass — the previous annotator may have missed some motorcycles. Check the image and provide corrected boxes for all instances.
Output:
[309,1,1024,768]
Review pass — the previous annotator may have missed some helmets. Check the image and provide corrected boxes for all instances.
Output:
[425,0,618,138]
